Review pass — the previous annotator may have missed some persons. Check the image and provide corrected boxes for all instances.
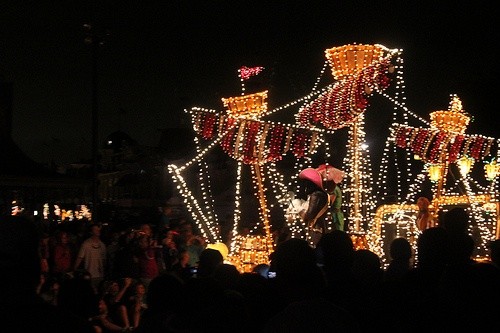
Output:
[15,202,500,333]
